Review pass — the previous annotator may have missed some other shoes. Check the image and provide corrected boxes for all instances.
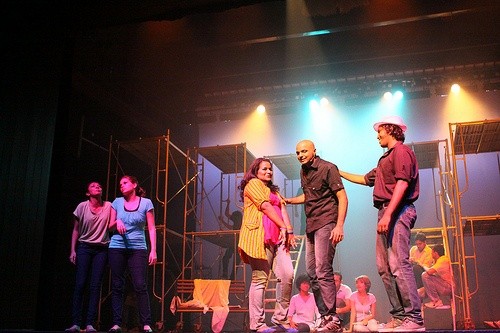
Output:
[249,326,272,333]
[109,327,123,333]
[87,327,97,333]
[144,327,153,333]
[417,287,425,295]
[378,318,404,332]
[64,327,81,332]
[394,321,427,331]
[272,317,292,330]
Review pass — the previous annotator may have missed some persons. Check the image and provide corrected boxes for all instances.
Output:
[407,232,435,297]
[222,198,243,281]
[342,275,387,333]
[331,271,353,328]
[338,115,426,332]
[279,140,349,333]
[421,244,456,310]
[287,274,322,329]
[237,158,301,333]
[63,181,113,333]
[106,174,158,333]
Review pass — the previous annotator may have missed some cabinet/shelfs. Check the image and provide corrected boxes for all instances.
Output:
[403,116,500,333]
[97,127,307,333]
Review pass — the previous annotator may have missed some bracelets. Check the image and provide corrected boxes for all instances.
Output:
[286,225,293,230]
[286,232,294,234]
[279,227,286,230]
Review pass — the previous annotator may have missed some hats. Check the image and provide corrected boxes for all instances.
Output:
[374,116,407,133]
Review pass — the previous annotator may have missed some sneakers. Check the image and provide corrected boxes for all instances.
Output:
[314,317,334,331]
[425,300,443,309]
[316,321,342,333]
